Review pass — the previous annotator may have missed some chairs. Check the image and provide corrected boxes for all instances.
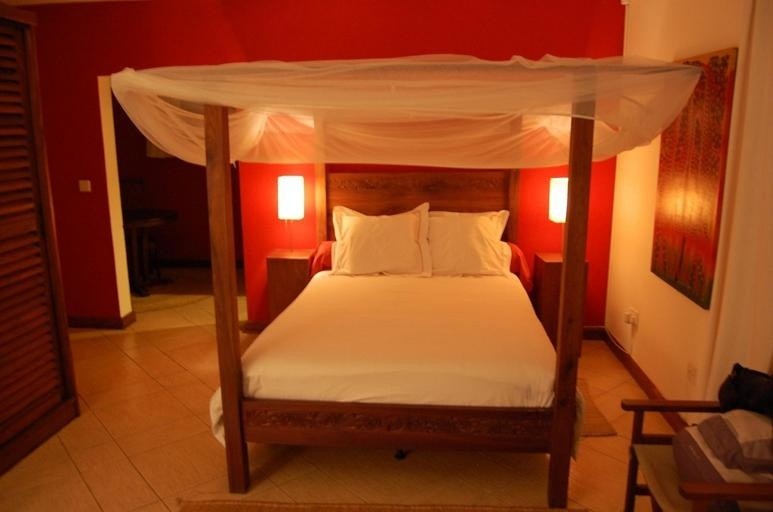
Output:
[619,395,772,511]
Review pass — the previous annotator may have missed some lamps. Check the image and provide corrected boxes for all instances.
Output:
[548,177,569,224]
[277,175,304,223]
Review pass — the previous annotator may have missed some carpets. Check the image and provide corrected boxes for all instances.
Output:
[576,377,616,437]
[172,502,587,512]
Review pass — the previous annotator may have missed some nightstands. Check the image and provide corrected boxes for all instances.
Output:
[534,252,589,347]
[266,250,313,321]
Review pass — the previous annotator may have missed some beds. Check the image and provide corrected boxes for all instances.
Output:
[213,163,585,462]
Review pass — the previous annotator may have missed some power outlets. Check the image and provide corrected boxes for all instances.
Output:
[625,308,640,325]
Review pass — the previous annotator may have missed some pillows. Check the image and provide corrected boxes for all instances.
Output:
[428,211,511,277]
[331,204,432,279]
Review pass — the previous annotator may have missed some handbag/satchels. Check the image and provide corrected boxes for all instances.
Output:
[716,363,772,417]
